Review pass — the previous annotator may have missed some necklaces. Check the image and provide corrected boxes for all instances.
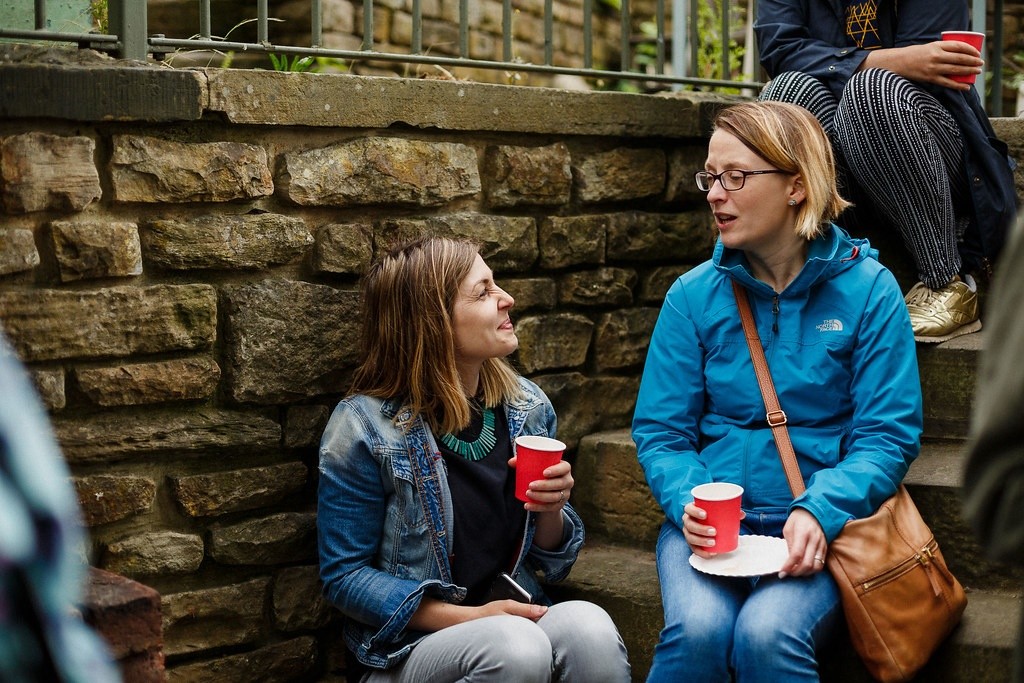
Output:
[434,403,497,462]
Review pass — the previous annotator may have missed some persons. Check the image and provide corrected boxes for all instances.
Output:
[314,236,633,683]
[749,1,1019,344]
[630,99,924,683]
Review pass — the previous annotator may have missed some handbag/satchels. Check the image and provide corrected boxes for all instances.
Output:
[826,484,972,682]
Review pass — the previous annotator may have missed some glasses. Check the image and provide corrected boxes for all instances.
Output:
[695,169,789,192]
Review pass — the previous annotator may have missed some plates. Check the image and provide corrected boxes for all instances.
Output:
[688,534,790,577]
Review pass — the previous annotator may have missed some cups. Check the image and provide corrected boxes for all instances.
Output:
[515,436,567,505]
[940,31,985,85]
[691,482,744,554]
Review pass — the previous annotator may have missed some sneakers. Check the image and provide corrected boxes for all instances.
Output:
[903,273,984,344]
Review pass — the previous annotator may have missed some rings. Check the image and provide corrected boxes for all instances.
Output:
[560,489,565,504]
[813,554,825,564]
